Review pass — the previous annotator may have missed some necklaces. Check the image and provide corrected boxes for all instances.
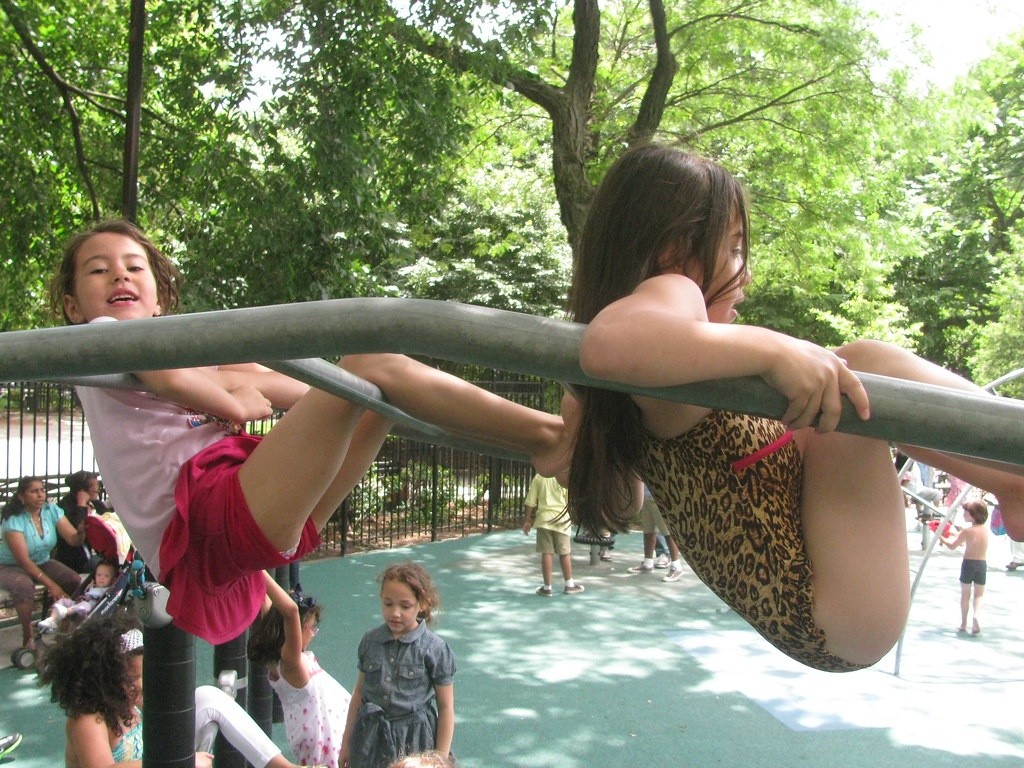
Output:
[34,517,43,537]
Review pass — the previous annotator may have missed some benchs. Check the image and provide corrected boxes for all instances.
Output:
[0,585,54,670]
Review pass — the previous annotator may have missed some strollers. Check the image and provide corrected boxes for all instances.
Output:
[10,511,146,670]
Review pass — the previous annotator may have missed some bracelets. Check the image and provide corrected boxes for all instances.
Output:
[35,572,44,582]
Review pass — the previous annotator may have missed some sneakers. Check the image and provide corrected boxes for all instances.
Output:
[626,560,654,574]
[536,586,553,596]
[662,565,684,582]
[655,554,672,569]
[601,551,612,561]
[564,583,585,593]
[0,733,23,760]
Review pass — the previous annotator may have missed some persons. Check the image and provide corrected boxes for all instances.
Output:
[386,750,455,768]
[37,559,119,628]
[51,218,646,646]
[54,470,114,574]
[1005,539,1024,570]
[599,484,684,581]
[338,562,458,768]
[522,473,585,597]
[247,570,353,768]
[545,141,1024,673]
[33,608,329,768]
[934,499,989,634]
[0,476,89,646]
[894,452,943,532]
